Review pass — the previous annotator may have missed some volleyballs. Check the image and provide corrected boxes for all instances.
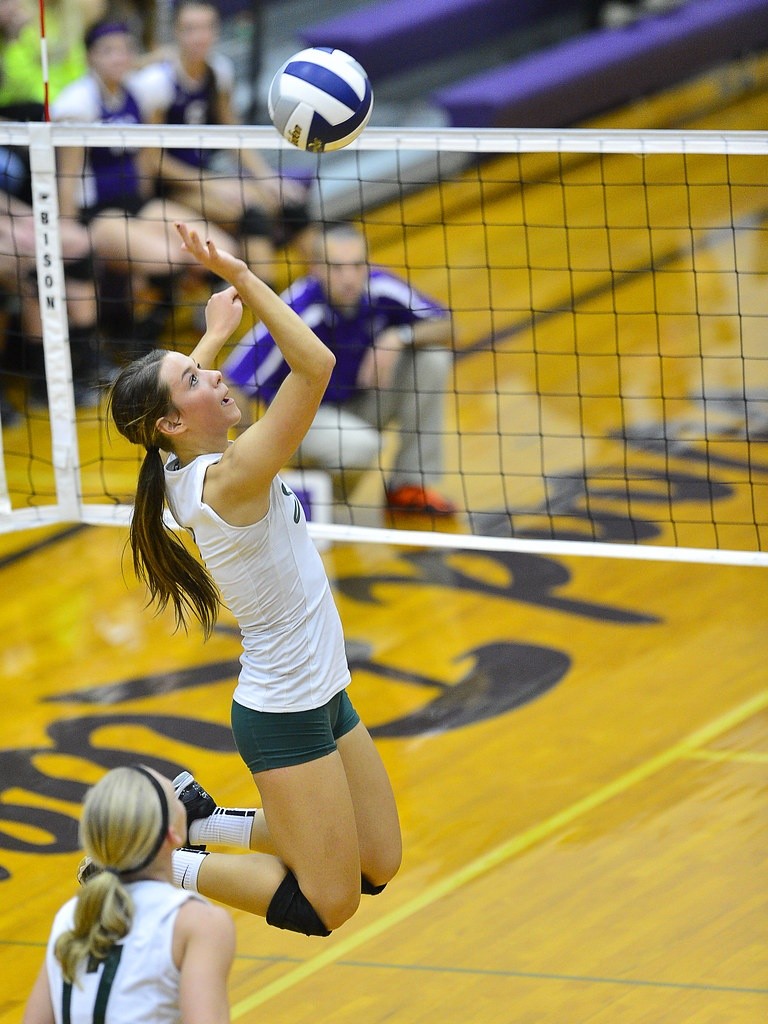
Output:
[266,46,375,155]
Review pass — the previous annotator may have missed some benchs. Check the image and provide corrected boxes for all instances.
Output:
[418,0,768,127]
[294,0,559,73]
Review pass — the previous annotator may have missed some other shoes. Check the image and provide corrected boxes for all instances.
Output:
[75,350,119,387]
[29,381,99,410]
[171,770,216,851]
[384,486,455,519]
[0,399,22,429]
[78,855,103,887]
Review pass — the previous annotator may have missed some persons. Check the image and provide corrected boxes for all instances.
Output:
[76,226,402,937]
[21,765,235,1024]
[221,228,458,517]
[0,0,322,424]
